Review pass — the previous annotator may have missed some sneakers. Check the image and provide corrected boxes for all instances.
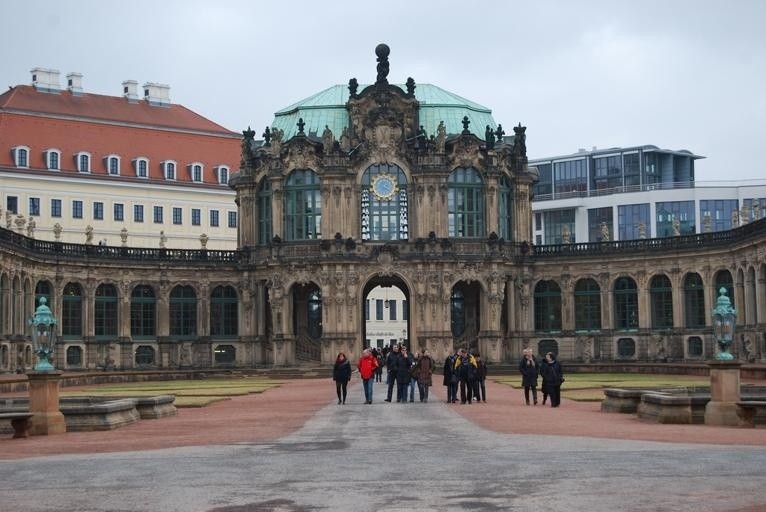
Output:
[338,399,345,404]
[384,397,428,403]
[363,400,373,404]
[526,401,545,406]
[447,397,486,404]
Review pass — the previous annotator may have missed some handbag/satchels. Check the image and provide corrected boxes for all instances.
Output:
[409,367,420,378]
[560,378,564,384]
[551,402,560,408]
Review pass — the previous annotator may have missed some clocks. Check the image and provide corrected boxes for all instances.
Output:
[369,171,399,201]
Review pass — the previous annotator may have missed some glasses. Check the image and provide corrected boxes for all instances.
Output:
[394,347,407,351]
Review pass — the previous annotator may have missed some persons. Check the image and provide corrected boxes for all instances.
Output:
[518,347,565,407]
[333,342,487,405]
